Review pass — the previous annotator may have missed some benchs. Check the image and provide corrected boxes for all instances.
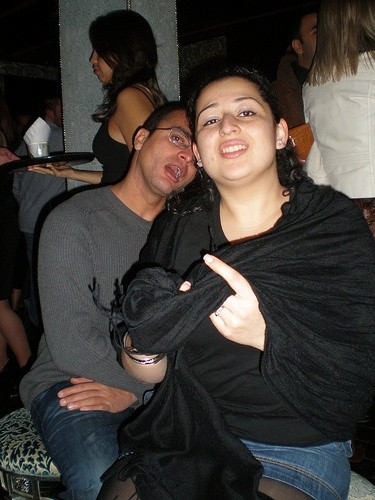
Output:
[0,408,375,500]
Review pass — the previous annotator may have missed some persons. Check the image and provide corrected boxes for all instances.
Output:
[28,10,168,186]
[18,100,197,500]
[301,0,375,198]
[269,6,317,130]
[1,91,68,399]
[91,65,375,500]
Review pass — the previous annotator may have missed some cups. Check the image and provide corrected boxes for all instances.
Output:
[28,143,49,158]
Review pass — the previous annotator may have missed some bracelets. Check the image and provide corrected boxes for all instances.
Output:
[122,331,167,365]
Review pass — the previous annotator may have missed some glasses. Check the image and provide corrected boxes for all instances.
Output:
[147,127,193,150]
